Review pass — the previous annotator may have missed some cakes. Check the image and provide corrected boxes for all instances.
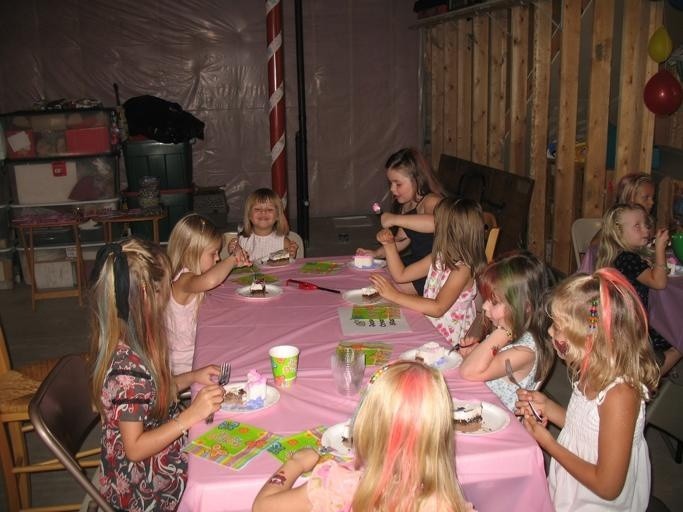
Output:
[268,249,290,267]
[451,397,483,432]
[415,341,445,365]
[245,368,268,401]
[361,285,379,303]
[250,278,266,297]
[353,256,373,268]
[340,418,352,448]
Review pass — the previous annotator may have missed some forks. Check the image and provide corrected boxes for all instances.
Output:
[314,442,336,456]
[205,360,230,425]
[504,358,544,424]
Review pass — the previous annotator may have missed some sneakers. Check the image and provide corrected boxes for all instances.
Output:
[670,364,680,384]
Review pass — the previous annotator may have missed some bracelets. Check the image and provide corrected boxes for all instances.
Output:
[174,417,187,436]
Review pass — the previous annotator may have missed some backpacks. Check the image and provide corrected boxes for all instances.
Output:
[123,95,204,144]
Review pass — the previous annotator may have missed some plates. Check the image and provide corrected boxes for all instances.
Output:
[661,262,682,278]
[255,256,294,268]
[346,257,388,271]
[319,420,357,459]
[233,283,284,300]
[449,397,510,436]
[342,288,382,305]
[399,348,463,372]
[216,382,281,413]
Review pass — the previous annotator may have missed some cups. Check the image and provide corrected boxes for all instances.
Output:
[329,351,365,394]
[265,345,301,381]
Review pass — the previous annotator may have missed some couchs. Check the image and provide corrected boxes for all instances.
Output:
[437,153,536,260]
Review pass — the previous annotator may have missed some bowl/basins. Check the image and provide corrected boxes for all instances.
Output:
[670,233,683,262]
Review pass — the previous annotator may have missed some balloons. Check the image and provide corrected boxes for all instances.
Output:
[648,26,673,64]
[644,71,683,115]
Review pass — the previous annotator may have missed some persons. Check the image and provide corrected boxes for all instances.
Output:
[228,189,299,262]
[577,173,656,274]
[594,202,682,377]
[459,248,556,423]
[251,360,478,512]
[163,212,252,376]
[85,236,226,512]
[356,147,449,296]
[514,266,662,512]
[369,196,488,349]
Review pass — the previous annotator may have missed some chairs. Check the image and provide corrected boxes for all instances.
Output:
[645,378,683,511]
[571,217,604,270]
[106,216,161,250]
[28,354,143,511]
[0,328,102,512]
[481,213,500,265]
[538,353,581,479]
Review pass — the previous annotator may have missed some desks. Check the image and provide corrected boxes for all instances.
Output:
[18,222,85,313]
